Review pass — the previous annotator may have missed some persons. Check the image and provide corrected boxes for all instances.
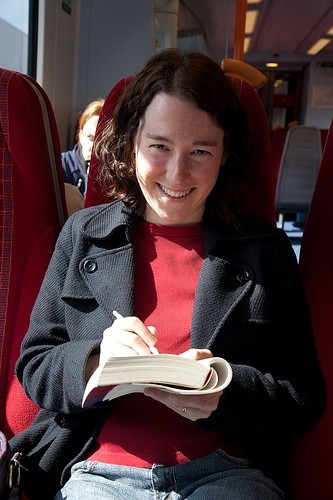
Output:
[60,98,105,199]
[0,47,328,500]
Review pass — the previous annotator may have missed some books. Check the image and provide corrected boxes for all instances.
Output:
[80,355,233,409]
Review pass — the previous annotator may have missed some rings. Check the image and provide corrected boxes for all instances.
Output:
[179,407,186,414]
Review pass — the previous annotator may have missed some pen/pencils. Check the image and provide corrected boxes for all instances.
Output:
[111,310,160,354]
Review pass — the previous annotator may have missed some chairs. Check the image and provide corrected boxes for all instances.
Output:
[0,68,68,441]
[288,117,332,499]
[82,75,277,228]
[270,125,328,213]
